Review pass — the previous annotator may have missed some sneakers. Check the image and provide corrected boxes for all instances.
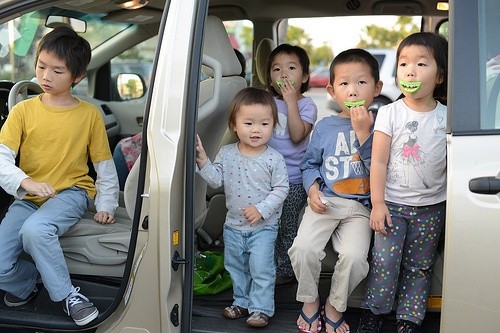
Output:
[224,305,249,319]
[246,311,269,327]
[4,277,43,307]
[62,285,99,326]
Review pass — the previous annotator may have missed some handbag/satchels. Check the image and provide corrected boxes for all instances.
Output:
[193,250,233,294]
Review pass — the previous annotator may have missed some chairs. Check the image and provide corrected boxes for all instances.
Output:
[57,15,277,277]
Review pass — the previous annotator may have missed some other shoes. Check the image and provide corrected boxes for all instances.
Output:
[355,309,384,333]
[276,278,297,289]
[388,319,420,333]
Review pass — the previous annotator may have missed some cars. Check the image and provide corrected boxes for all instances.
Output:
[309,65,332,88]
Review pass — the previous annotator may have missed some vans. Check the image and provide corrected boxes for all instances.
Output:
[327,48,404,122]
[0,0,499,333]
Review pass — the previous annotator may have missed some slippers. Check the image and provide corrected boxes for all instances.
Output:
[321,305,350,333]
[298,306,322,333]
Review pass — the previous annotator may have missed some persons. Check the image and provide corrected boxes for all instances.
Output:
[0,25,119,325]
[358,32,447,333]
[113,48,246,191]
[287,49,382,333]
[264,44,318,284]
[196,87,289,326]
